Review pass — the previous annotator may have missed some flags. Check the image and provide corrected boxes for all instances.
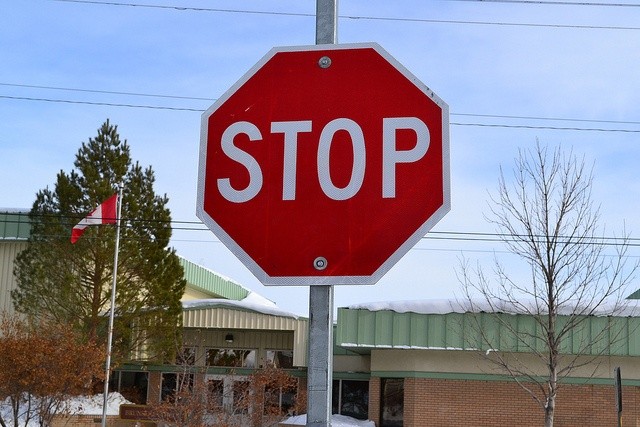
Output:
[71,194,118,244]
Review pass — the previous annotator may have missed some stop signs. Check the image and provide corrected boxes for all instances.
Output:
[196,41,451,285]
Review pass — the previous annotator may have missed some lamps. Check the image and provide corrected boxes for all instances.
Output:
[225,334,233,342]
